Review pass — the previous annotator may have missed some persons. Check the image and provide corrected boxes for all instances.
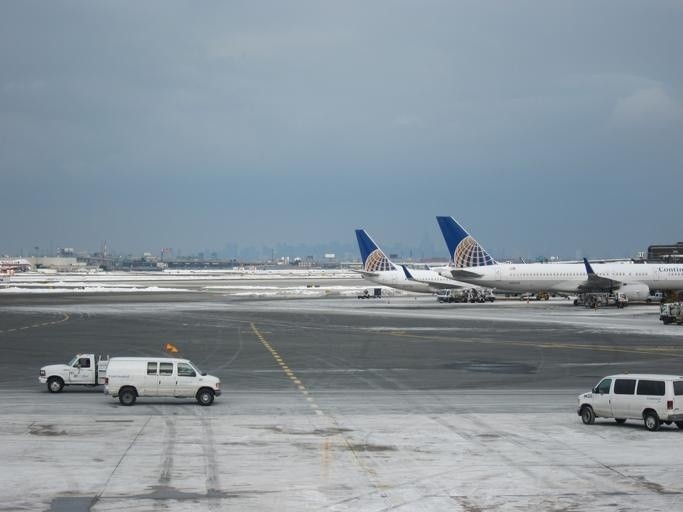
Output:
[81,360,89,367]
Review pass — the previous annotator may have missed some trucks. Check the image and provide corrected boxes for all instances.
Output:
[39,353,109,393]
[659,302,683,324]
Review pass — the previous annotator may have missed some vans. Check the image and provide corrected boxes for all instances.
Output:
[104,357,221,406]
[577,373,683,431]
